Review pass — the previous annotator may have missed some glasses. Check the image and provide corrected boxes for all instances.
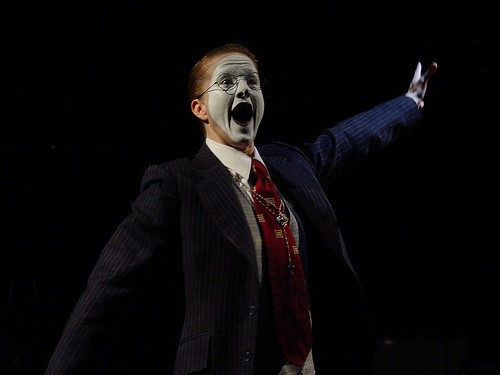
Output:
[196,72,264,98]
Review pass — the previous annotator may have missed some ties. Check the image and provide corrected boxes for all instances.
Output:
[248,159,313,367]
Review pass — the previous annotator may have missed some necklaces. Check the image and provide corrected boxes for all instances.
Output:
[232,172,298,274]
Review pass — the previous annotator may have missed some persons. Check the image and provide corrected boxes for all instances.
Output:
[47,42,438,375]
